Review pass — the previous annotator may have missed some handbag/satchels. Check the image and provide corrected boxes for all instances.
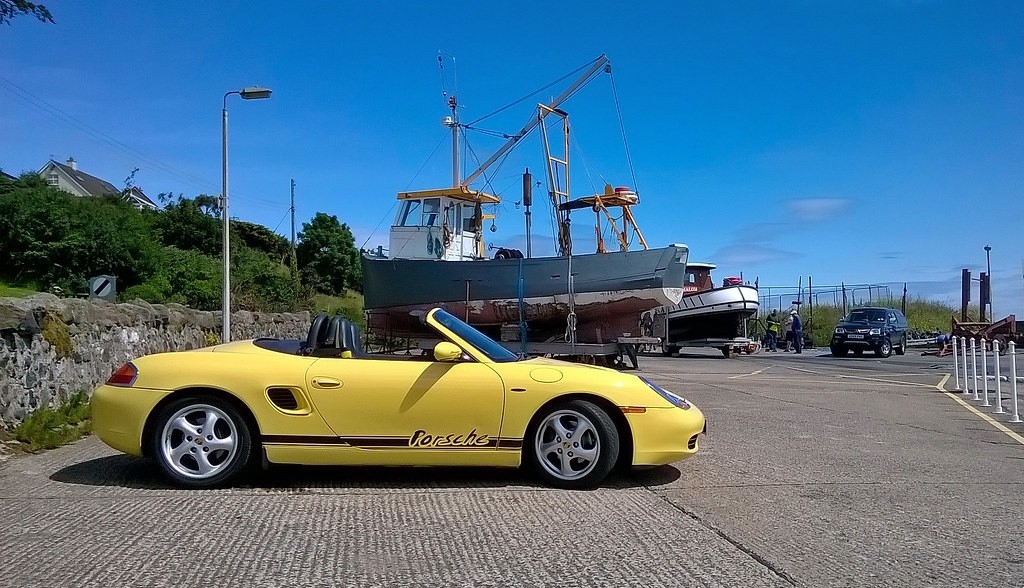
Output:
[769,323,777,334]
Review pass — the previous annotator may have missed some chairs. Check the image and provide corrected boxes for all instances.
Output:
[853,313,866,321]
[307,313,373,360]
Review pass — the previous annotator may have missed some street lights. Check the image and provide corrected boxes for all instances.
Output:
[221,87,272,344]
[984,244,993,323]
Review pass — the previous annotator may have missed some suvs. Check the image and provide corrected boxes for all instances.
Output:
[830,307,909,358]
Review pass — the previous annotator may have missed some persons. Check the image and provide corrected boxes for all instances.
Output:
[784,316,794,353]
[765,309,780,353]
[790,310,802,354]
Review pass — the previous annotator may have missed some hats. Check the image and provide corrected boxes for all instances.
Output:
[773,309,778,312]
[790,310,797,314]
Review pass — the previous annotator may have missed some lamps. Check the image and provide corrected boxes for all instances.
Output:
[489,202,497,232]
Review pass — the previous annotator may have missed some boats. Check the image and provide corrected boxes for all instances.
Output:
[641,263,761,341]
[359,49,689,344]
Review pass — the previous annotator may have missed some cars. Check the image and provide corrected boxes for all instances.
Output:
[89,307,707,490]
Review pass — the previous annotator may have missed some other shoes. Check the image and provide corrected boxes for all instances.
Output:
[794,352,801,354]
[783,350,790,352]
